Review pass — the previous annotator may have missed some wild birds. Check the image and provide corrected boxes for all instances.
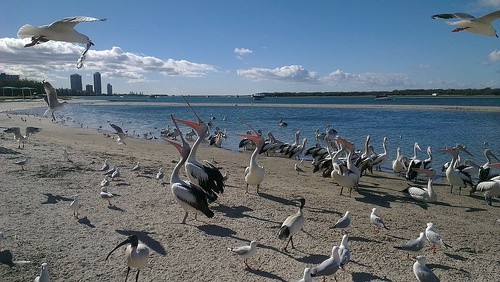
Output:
[106,233,149,282]
[305,125,500,206]
[99,157,120,200]
[299,208,447,282]
[13,158,27,166]
[431,10,500,38]
[3,127,42,149]
[17,16,107,69]
[156,168,164,180]
[228,240,258,264]
[33,262,50,282]
[133,162,141,172]
[34,82,68,121]
[235,134,265,194]
[278,198,306,253]
[161,95,229,225]
[0,110,89,128]
[109,124,127,145]
[63,148,69,160]
[96,115,307,174]
[69,194,80,215]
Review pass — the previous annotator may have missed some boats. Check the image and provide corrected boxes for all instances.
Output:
[432,93,439,96]
[376,93,387,98]
[253,95,265,100]
